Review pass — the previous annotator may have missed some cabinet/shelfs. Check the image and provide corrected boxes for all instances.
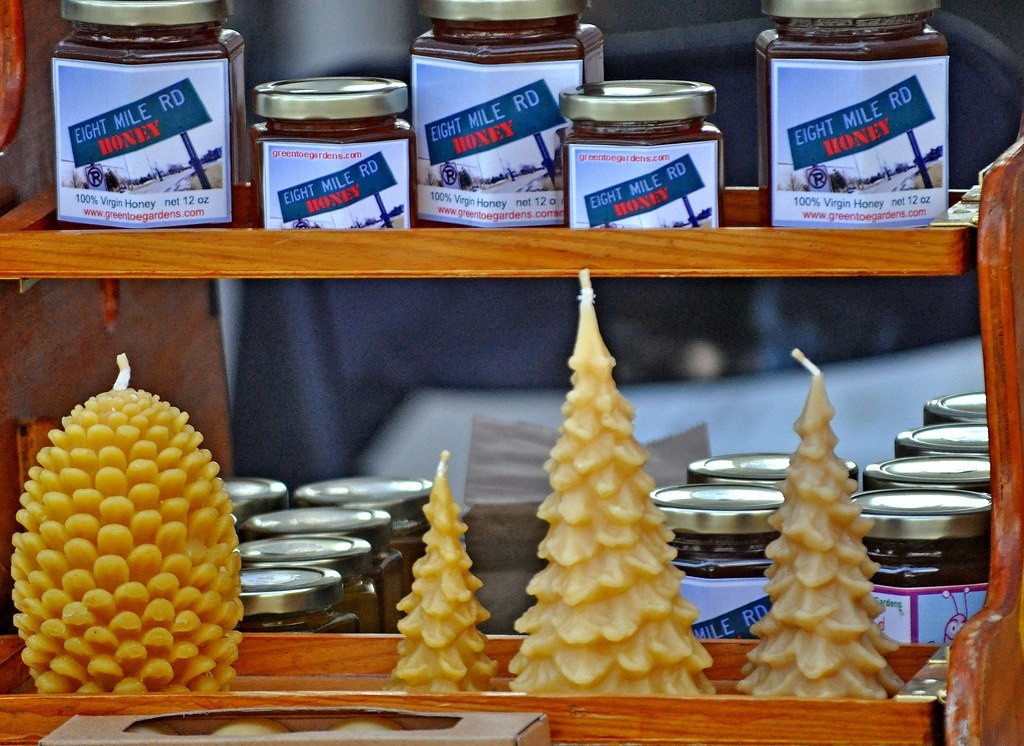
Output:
[0,128,1024,746]
